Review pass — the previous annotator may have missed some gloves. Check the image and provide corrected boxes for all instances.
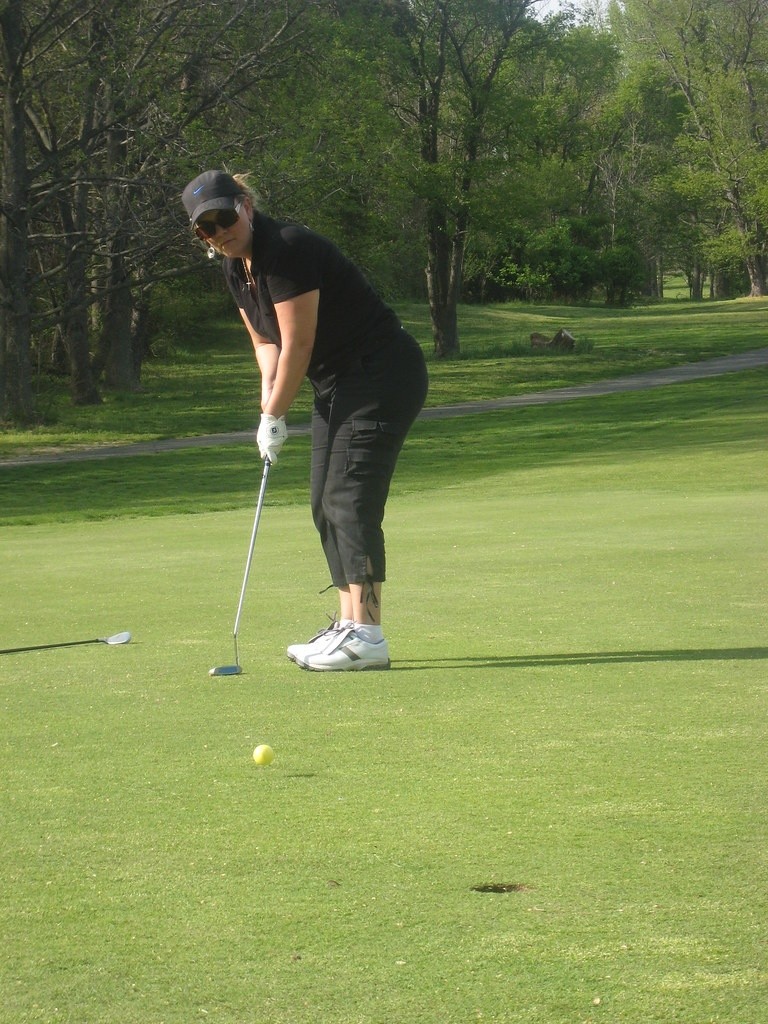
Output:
[256,413,288,466]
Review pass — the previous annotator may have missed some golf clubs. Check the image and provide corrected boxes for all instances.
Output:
[0,631,132,654]
[208,454,271,676]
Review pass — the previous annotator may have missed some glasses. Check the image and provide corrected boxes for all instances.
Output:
[194,199,243,240]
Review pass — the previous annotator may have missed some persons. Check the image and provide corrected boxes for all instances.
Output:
[183,169,429,672]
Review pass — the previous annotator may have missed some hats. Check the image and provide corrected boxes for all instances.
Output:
[181,170,245,230]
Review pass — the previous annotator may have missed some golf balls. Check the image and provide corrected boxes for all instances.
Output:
[253,744,274,765]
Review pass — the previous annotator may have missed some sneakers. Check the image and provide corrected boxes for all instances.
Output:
[286,611,391,671]
